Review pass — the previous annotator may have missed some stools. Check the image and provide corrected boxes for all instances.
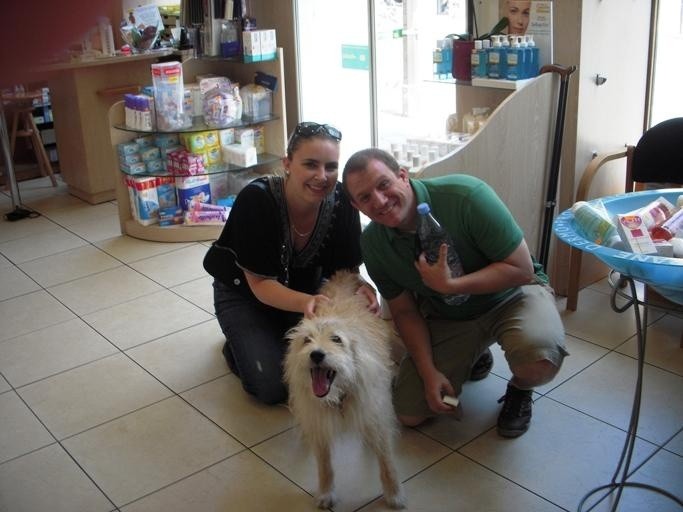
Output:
[0,92,57,191]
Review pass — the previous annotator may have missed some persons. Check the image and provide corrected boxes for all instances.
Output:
[342,148,570,437]
[506,0,531,36]
[203,123,383,405]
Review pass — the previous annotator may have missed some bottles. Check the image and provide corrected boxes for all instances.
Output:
[652,206,683,241]
[417,203,470,306]
[433,34,491,81]
[124,93,153,132]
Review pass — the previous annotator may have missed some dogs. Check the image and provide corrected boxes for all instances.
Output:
[281,270,407,511]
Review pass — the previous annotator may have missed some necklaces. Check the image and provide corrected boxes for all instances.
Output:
[287,203,318,238]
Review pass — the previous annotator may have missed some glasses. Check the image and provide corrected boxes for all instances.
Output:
[287,121,343,149]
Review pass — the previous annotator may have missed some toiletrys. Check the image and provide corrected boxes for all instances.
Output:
[485,35,538,79]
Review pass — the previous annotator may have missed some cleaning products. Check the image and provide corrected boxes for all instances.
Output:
[432,38,489,77]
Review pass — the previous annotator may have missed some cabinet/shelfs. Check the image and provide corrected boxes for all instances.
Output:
[113,112,283,240]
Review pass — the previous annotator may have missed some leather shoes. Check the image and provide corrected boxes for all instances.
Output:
[468,346,494,382]
[496,383,534,438]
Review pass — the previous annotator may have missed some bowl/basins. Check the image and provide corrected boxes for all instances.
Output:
[120,33,159,54]
